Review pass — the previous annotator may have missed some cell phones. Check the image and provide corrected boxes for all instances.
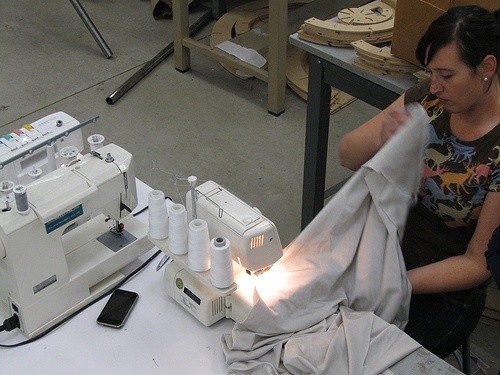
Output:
[96,289,139,328]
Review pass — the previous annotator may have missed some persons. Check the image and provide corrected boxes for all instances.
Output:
[338,6,499,359]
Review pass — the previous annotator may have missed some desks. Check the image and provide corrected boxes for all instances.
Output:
[0,177,465,375]
[288,0,418,233]
